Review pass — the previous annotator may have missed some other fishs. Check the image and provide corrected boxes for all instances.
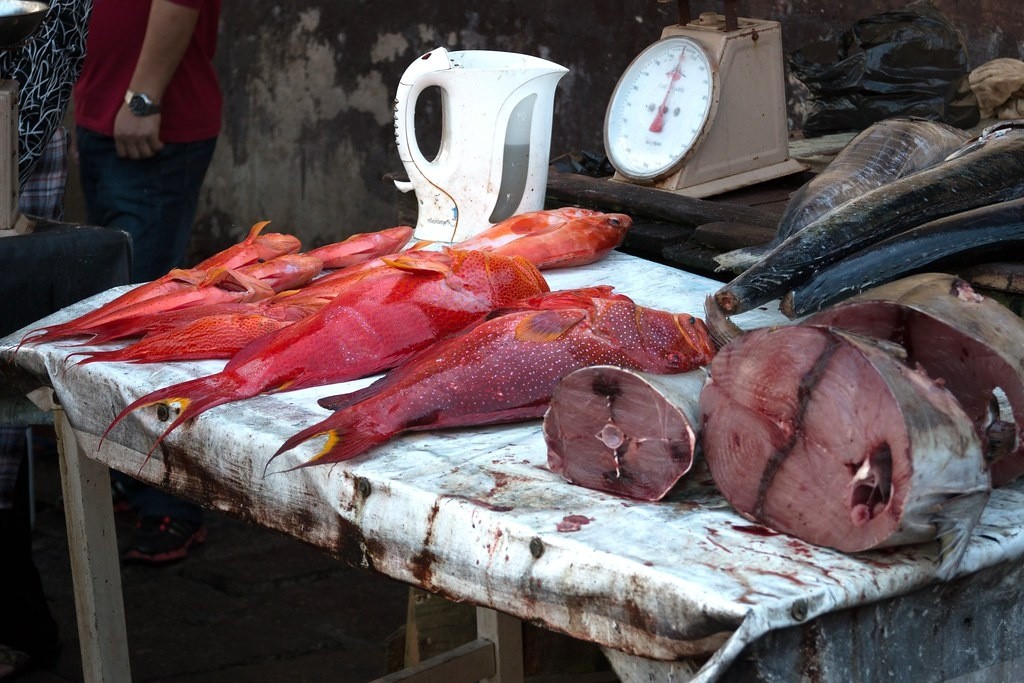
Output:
[11,115,1024,583]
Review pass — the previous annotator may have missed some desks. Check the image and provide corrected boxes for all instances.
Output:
[0,249,1024,683]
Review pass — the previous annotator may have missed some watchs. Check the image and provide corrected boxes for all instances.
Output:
[125,89,160,115]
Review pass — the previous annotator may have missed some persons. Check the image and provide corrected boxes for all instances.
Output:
[73,0,223,564]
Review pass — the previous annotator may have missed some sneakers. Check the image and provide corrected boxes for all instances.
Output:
[121,514,209,563]
[54,475,136,513]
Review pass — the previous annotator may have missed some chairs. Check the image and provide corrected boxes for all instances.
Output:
[0,228,139,529]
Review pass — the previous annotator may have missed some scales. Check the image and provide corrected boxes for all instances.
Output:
[601,1,811,197]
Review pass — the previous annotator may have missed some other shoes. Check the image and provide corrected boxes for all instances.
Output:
[0,636,63,683]
